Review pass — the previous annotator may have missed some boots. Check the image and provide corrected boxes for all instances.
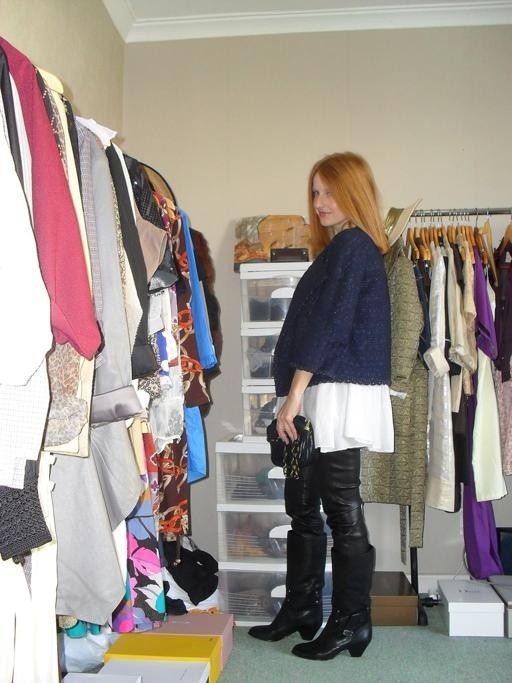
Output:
[247,528,328,642]
[290,543,376,660]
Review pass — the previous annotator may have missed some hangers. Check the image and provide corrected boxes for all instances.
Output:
[408,207,512,285]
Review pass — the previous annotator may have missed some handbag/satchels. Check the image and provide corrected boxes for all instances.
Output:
[266,415,315,481]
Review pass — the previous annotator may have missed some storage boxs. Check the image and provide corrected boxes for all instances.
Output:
[217,504,293,564]
[240,379,277,442]
[238,261,313,320]
[61,612,235,683]
[241,321,285,379]
[214,442,286,506]
[217,561,288,626]
[367,566,512,641]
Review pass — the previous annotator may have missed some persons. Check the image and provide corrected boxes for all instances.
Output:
[249,153,394,660]
